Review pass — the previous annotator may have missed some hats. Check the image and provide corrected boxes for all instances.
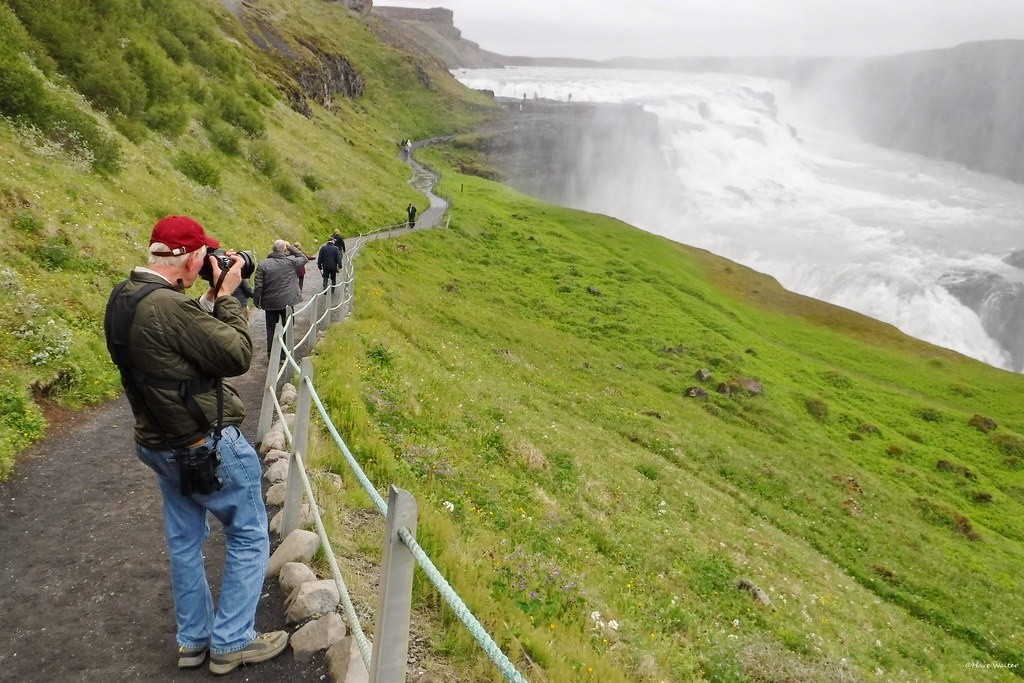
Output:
[148,215,220,257]
[327,237,336,242]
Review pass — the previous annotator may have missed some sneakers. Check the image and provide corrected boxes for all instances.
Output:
[177,645,208,668]
[280,359,285,363]
[210,630,288,675]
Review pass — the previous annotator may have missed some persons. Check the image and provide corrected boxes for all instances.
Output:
[407,204,416,228]
[333,229,346,272]
[253,240,308,364]
[400,139,412,160]
[293,242,316,290]
[317,238,342,295]
[103,217,288,674]
[230,278,254,322]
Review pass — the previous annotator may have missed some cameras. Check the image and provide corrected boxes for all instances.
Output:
[198,249,255,286]
